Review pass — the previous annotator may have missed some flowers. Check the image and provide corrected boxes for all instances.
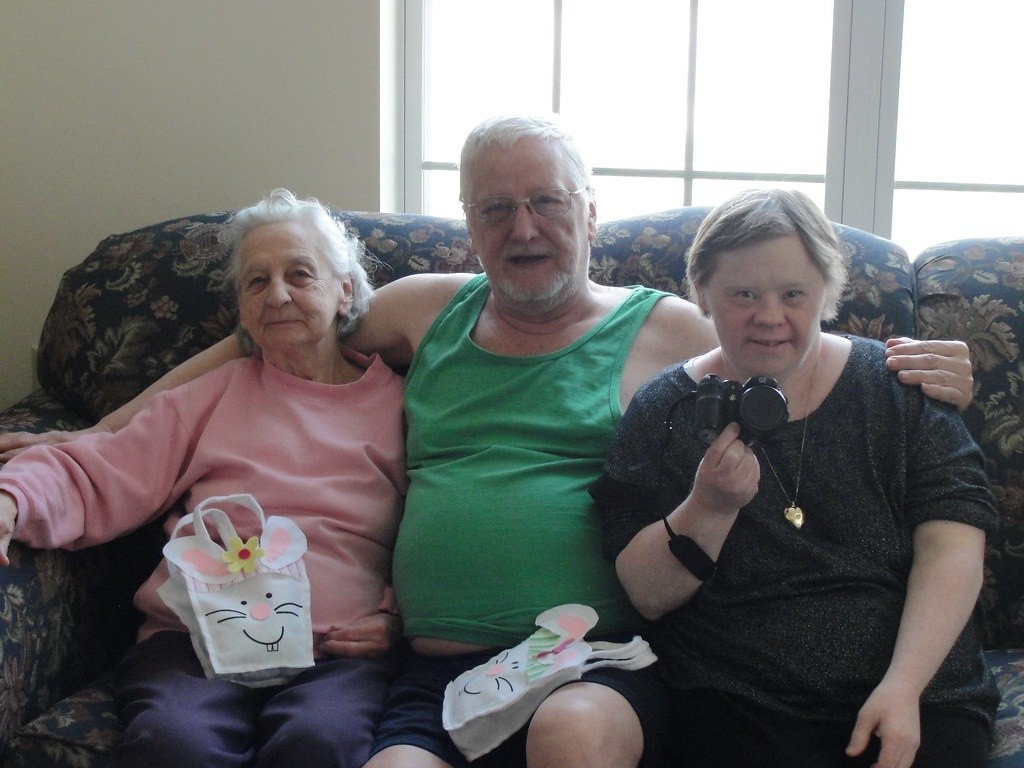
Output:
[222,536,263,573]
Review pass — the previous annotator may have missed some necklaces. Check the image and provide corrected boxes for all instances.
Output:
[718,349,821,528]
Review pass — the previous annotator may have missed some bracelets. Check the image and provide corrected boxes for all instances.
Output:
[662,516,720,583]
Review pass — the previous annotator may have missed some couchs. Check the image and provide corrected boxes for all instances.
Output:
[0,208,1024,768]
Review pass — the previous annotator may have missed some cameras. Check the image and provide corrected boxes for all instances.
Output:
[697,373,790,447]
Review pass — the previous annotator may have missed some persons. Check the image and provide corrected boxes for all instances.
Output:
[0,187,406,768]
[586,189,1003,768]
[0,113,973,768]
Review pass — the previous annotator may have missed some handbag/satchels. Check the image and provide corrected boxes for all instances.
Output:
[442,604,658,763]
[158,493,316,687]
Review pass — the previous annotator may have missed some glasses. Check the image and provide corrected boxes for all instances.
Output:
[467,188,588,225]
